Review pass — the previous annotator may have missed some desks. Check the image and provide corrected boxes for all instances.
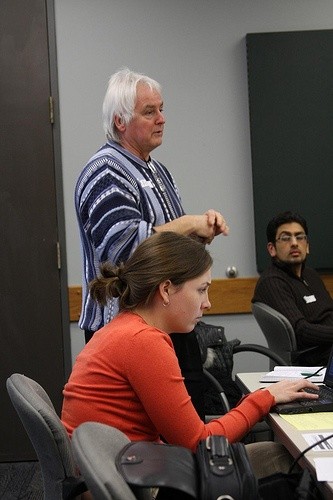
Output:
[236,372,333,500]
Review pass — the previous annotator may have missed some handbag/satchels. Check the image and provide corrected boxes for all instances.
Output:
[192,319,241,411]
[115,432,258,500]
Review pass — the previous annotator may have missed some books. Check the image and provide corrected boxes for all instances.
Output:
[258,365,327,384]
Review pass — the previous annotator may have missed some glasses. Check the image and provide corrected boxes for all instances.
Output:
[274,235,308,244]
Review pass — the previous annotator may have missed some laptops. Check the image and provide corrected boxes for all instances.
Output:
[274,359,333,414]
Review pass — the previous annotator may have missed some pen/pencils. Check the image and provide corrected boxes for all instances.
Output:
[301,372,323,376]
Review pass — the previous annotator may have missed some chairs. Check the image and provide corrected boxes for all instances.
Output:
[71,421,153,500]
[200,299,330,443]
[6,373,86,500]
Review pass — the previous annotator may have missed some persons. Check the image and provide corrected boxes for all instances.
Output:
[250,210,333,380]
[56,231,320,500]
[73,67,230,345]
